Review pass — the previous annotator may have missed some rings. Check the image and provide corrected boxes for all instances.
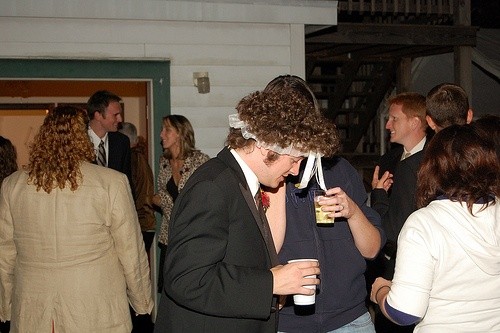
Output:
[340,204,344,210]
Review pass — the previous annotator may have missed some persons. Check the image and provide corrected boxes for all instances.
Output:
[118,121,157,252]
[0,105,154,333]
[366,82,473,333]
[0,135,18,186]
[154,87,341,333]
[371,122,500,333]
[80,90,132,186]
[152,114,209,294]
[258,75,387,333]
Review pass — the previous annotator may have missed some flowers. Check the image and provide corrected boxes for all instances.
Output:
[259,188,271,212]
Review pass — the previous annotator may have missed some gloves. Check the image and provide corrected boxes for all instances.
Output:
[130,314,154,333]
[0,319,10,333]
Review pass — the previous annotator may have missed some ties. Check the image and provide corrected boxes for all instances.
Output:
[97,140,106,166]
[254,187,265,228]
[400,152,411,160]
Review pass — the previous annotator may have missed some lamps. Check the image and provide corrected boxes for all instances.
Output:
[193,71,210,94]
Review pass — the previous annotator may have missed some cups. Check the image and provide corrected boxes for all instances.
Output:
[314,190,336,223]
[288,259,318,305]
